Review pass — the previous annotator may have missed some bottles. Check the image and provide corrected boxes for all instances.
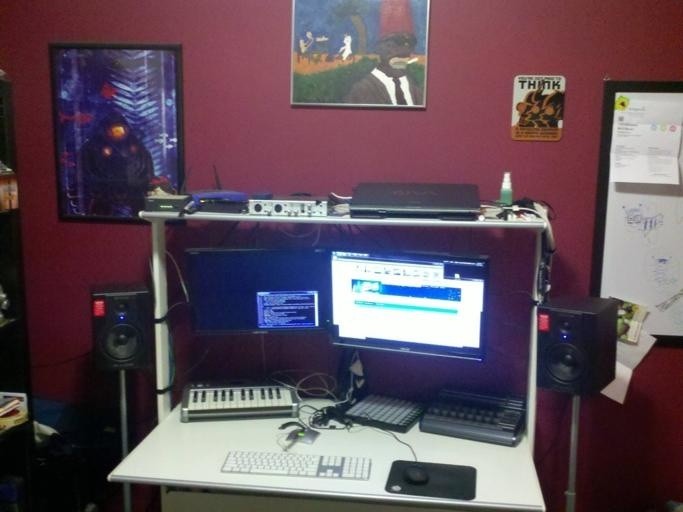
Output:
[500,172,513,207]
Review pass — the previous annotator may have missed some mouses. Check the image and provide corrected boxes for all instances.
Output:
[402,464,428,483]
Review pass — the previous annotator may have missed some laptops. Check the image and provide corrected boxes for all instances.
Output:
[348,181,482,220]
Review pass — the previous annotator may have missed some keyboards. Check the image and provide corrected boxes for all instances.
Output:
[222,450,372,481]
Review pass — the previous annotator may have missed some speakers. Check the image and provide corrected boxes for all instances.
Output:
[89,288,152,372]
[536,298,620,393]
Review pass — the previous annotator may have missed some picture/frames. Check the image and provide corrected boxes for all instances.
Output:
[46,38,185,226]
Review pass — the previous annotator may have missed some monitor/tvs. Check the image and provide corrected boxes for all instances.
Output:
[184,246,331,334]
[331,249,490,365]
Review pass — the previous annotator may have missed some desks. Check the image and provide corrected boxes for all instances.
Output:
[108,398,552,512]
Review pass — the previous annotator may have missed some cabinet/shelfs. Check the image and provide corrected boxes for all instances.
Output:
[0,72,45,512]
